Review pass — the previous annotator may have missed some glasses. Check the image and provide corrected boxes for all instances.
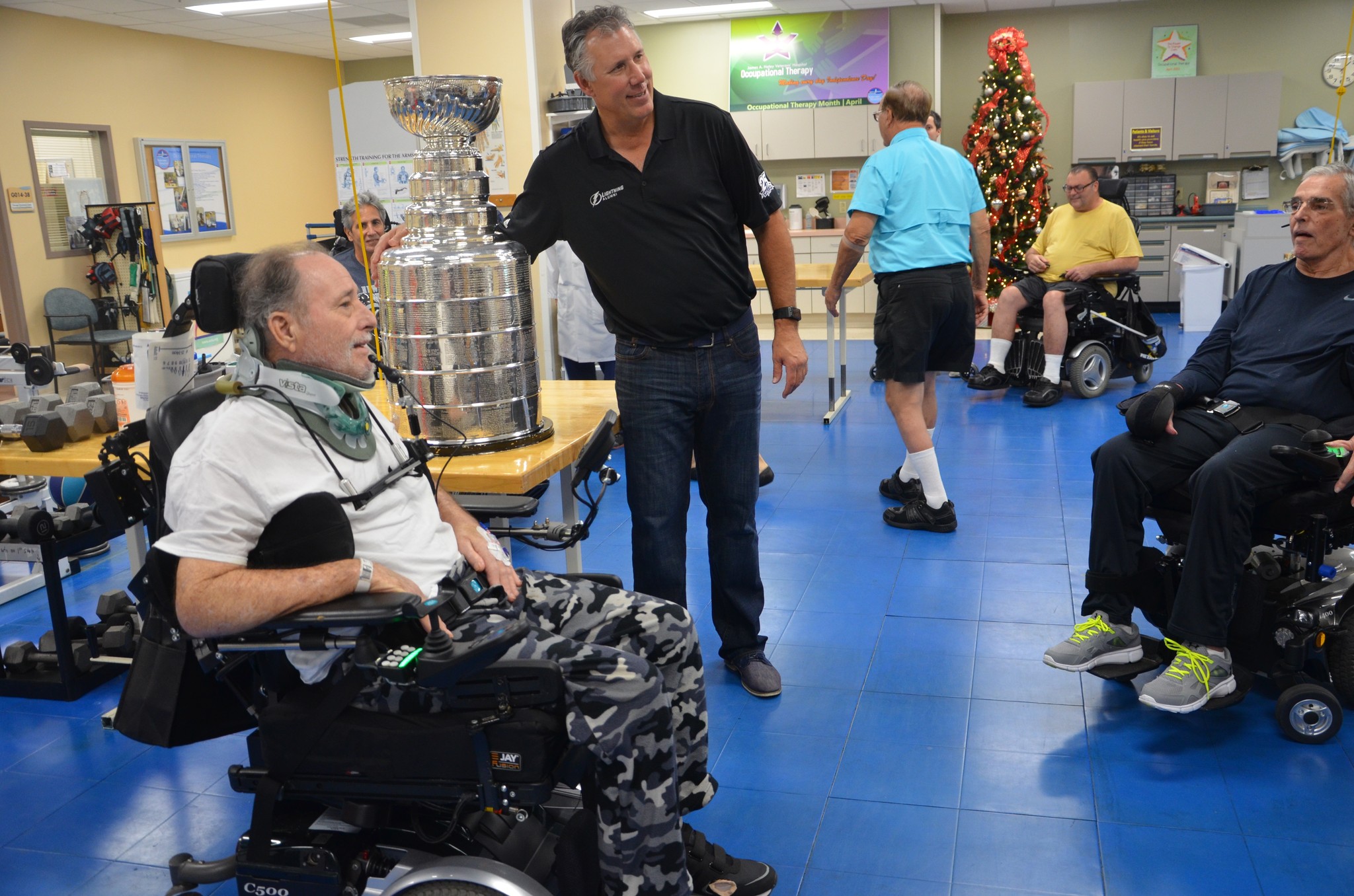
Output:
[1062,179,1096,193]
[873,109,893,122]
[1281,198,1354,214]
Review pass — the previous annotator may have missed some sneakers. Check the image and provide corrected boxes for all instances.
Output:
[967,364,1010,390]
[1139,638,1236,714]
[879,466,925,505]
[724,650,782,697]
[1023,377,1063,406]
[1042,610,1143,672]
[680,823,777,896]
[882,493,958,534]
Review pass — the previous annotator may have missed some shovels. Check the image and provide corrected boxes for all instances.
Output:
[815,196,831,219]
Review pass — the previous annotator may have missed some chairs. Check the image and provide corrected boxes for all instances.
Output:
[43,287,139,395]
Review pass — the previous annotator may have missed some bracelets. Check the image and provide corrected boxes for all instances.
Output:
[355,558,373,595]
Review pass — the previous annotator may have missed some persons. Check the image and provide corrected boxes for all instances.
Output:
[150,240,776,896]
[967,165,1143,407]
[338,192,773,488]
[1043,162,1354,714]
[825,79,991,533]
[371,8,808,696]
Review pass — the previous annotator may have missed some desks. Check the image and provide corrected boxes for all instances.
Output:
[0,380,622,573]
[749,262,875,427]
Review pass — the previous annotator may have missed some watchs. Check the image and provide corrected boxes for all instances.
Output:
[773,306,802,322]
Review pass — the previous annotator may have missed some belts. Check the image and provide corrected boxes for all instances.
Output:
[623,306,753,347]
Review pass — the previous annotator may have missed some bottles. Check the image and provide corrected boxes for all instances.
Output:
[194,352,211,374]
[789,204,803,230]
[806,212,812,230]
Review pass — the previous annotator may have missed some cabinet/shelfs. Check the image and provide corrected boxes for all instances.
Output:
[1071,72,1282,165]
[1115,215,1235,304]
[731,104,888,161]
[742,236,879,316]
[0,513,148,702]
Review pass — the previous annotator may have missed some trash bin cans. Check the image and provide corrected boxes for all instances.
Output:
[1176,242,1230,332]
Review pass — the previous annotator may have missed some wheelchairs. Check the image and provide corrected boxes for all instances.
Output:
[98,371,626,896]
[1078,388,1354,746]
[1004,174,1155,405]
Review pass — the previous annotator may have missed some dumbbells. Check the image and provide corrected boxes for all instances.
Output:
[60,612,134,634]
[0,410,69,454]
[6,501,93,539]
[0,399,96,443]
[95,587,150,623]
[37,624,134,659]
[65,379,105,405]
[3,641,93,675]
[0,508,56,544]
[27,393,118,433]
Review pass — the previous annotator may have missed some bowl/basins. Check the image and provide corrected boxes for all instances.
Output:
[816,218,834,229]
[193,363,224,389]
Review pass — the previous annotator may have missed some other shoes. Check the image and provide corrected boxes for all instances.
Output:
[612,432,624,448]
[690,467,698,481]
[757,465,775,486]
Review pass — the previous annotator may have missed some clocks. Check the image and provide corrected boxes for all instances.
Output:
[1322,51,1354,88]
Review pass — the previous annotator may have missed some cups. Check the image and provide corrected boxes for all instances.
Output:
[111,364,144,429]
